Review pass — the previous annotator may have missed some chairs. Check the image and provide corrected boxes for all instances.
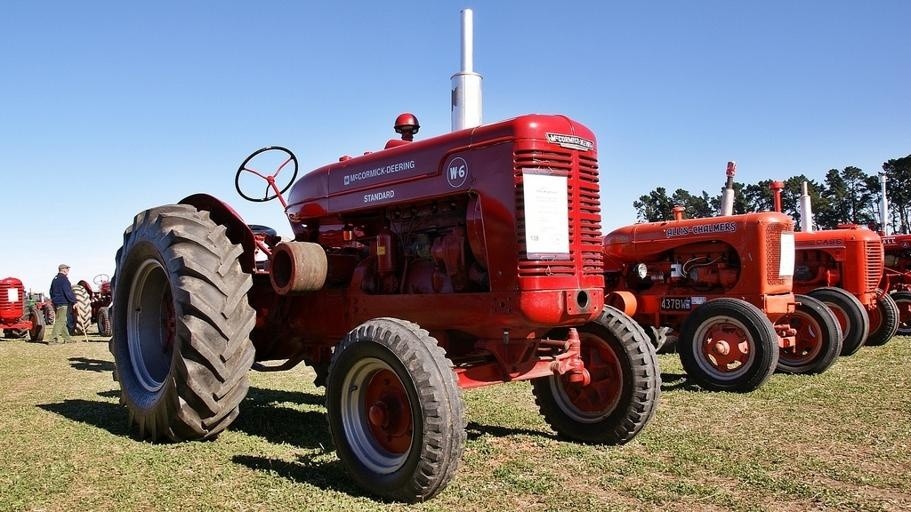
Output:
[248,224,273,242]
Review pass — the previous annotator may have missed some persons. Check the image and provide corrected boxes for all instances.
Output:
[48,261,79,345]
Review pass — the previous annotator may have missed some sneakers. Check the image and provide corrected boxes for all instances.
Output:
[47,339,77,345]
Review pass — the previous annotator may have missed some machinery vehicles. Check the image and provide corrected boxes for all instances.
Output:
[0,274,112,343]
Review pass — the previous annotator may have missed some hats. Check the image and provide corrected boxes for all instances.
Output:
[58,263,71,270]
